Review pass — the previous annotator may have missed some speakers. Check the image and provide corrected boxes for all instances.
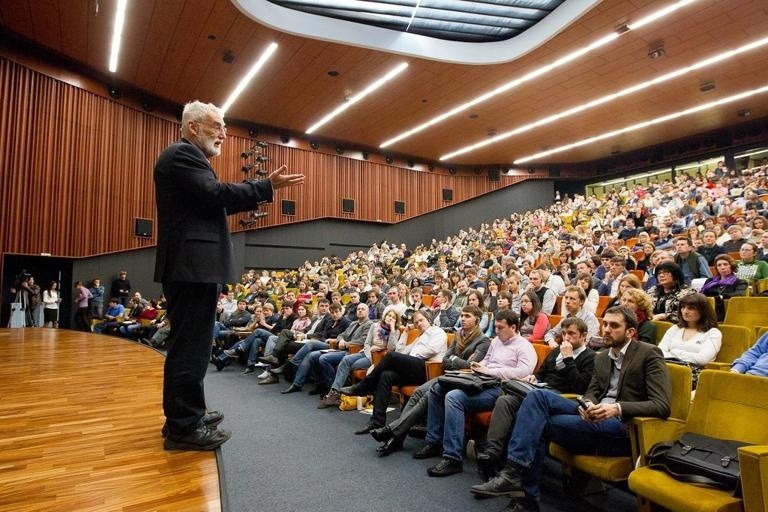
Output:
[343,198,354,213]
[395,200,405,215]
[281,199,296,216]
[443,188,452,201]
[488,170,499,182]
[549,164,560,176]
[134,217,153,238]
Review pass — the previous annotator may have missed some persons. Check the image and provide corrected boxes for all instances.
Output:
[74,270,171,349]
[210,162,768,496]
[22,276,64,329]
[155,100,306,451]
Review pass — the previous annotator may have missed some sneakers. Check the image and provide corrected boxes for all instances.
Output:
[241,367,254,375]
[212,354,224,371]
[223,349,240,358]
[142,338,154,346]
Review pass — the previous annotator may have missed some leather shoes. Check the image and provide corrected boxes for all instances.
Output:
[162,411,224,436]
[163,420,231,450]
[254,354,368,409]
[355,417,540,511]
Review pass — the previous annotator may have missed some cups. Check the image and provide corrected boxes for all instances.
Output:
[295,334,302,342]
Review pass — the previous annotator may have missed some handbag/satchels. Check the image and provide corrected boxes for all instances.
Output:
[664,357,704,390]
[438,371,499,397]
[666,431,756,492]
[504,379,562,402]
